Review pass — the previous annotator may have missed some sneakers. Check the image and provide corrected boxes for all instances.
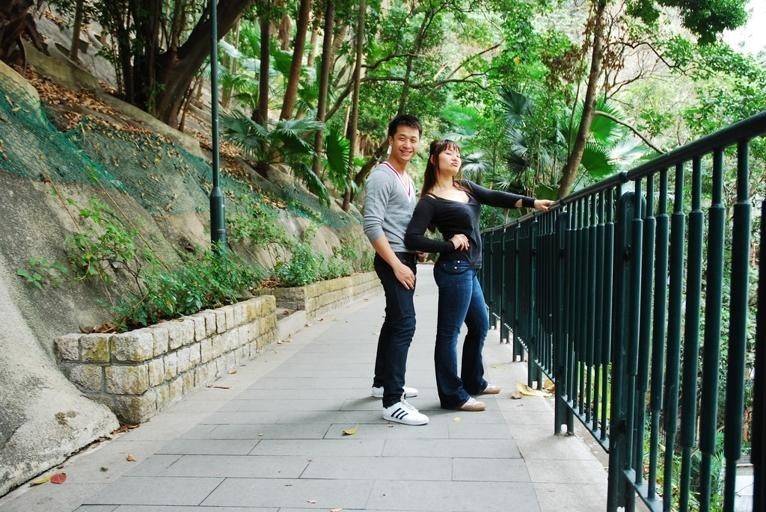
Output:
[442,395,486,410]
[476,383,502,395]
[371,385,419,399]
[380,400,430,427]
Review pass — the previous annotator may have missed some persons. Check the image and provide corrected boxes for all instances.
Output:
[404,139,555,411]
[363,114,429,425]
